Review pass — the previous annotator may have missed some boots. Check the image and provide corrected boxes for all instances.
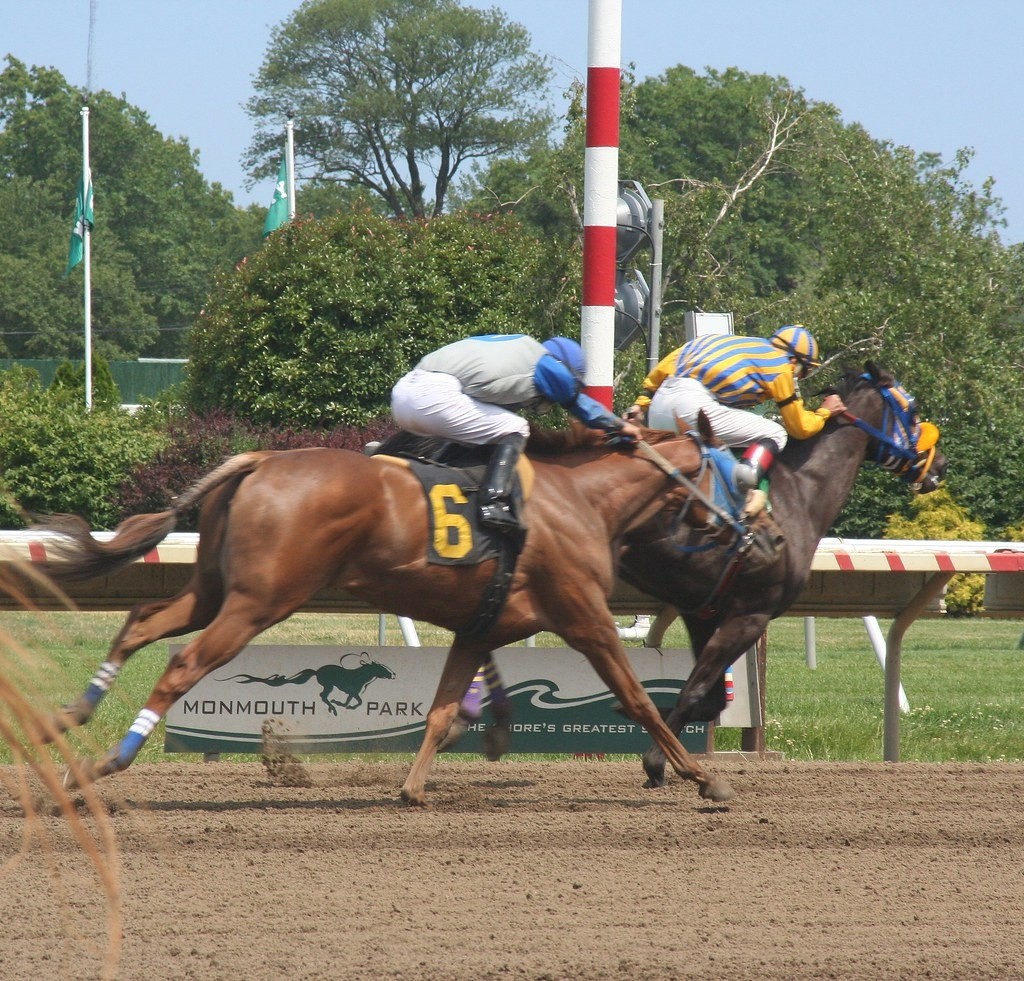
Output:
[737,438,778,498]
[478,433,529,534]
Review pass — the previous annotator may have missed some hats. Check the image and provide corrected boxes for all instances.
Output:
[542,337,586,388]
[768,324,835,372]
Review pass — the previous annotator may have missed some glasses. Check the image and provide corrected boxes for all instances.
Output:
[799,365,808,381]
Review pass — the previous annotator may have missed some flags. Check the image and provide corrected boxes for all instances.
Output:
[65,135,94,273]
[260,145,289,238]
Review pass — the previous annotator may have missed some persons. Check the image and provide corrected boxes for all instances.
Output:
[622,324,849,494]
[367,334,645,534]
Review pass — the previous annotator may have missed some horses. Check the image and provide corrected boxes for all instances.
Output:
[27,409,788,813]
[461,361,946,801]
